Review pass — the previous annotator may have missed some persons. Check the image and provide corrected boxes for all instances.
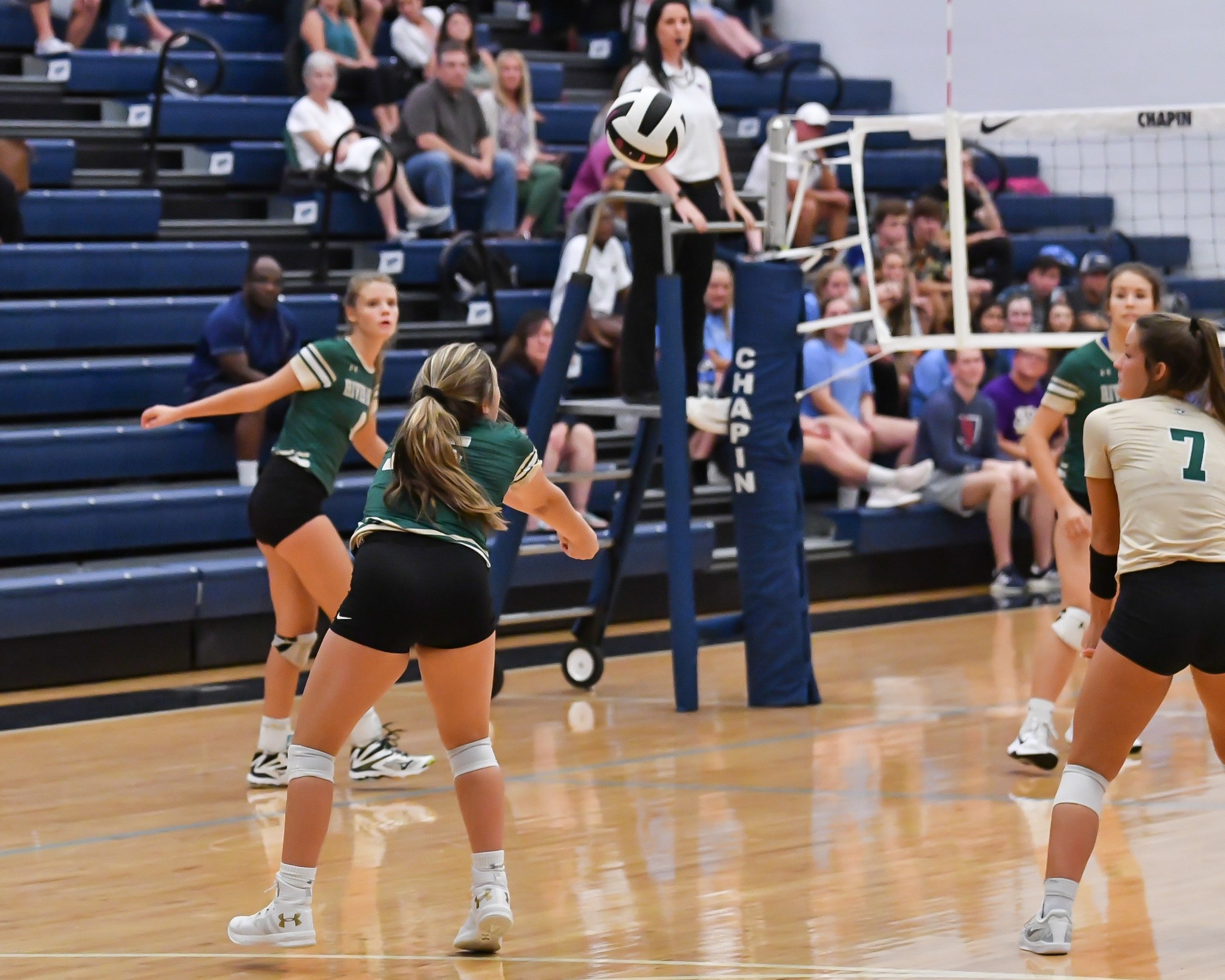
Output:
[0,0,1225,954]
[140,269,435,787]
[228,343,599,953]
[182,252,304,487]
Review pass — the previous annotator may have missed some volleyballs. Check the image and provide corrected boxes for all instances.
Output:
[604,88,687,168]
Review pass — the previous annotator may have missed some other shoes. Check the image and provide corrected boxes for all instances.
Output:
[988,563,1026,588]
[34,36,74,58]
[406,205,452,231]
[1026,557,1061,580]
[387,230,418,243]
[866,486,921,509]
[109,46,143,57]
[147,34,190,54]
[895,459,934,494]
[1064,720,1143,753]
[1006,711,1060,771]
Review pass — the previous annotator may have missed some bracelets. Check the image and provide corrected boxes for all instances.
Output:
[673,190,685,205]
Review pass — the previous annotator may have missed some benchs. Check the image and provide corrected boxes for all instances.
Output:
[0,0,1225,650]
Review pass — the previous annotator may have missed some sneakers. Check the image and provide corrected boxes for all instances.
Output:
[1018,908,1071,954]
[227,875,316,949]
[348,721,436,781]
[246,749,289,787]
[453,864,514,952]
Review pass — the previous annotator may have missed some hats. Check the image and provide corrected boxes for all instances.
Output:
[1079,249,1112,274]
[794,101,831,126]
